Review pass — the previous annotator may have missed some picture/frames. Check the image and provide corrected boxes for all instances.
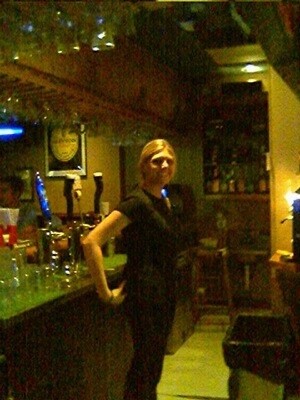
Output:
[13,167,35,202]
[43,120,87,177]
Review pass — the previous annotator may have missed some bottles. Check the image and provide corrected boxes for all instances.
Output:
[0,243,79,315]
[205,166,269,193]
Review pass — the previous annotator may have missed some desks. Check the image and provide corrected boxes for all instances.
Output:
[229,246,269,292]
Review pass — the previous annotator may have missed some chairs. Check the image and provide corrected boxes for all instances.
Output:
[190,212,236,326]
[223,268,300,400]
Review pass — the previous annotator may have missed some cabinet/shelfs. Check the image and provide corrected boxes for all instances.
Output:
[200,92,270,202]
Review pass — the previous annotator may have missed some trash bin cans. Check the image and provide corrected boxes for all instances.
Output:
[223,313,291,400]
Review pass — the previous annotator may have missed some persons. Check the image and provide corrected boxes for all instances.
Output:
[82,138,182,400]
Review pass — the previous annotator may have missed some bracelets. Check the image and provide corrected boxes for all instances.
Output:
[108,290,114,304]
